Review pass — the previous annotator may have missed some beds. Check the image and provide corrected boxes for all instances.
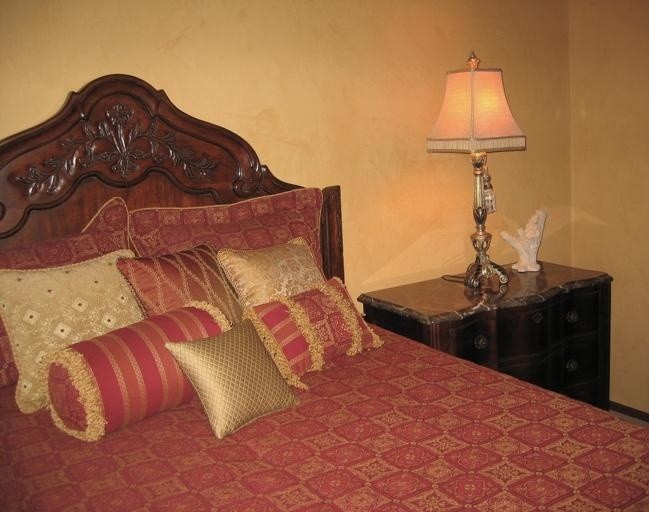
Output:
[1,72,649,512]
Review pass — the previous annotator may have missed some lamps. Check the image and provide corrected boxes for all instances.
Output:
[426,52,526,288]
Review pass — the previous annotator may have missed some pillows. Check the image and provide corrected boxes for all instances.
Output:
[1,249,163,414]
[218,239,330,319]
[248,278,381,385]
[48,302,236,442]
[2,195,131,383]
[127,186,324,275]
[164,322,303,440]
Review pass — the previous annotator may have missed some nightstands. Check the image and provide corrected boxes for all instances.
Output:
[357,259,614,414]
